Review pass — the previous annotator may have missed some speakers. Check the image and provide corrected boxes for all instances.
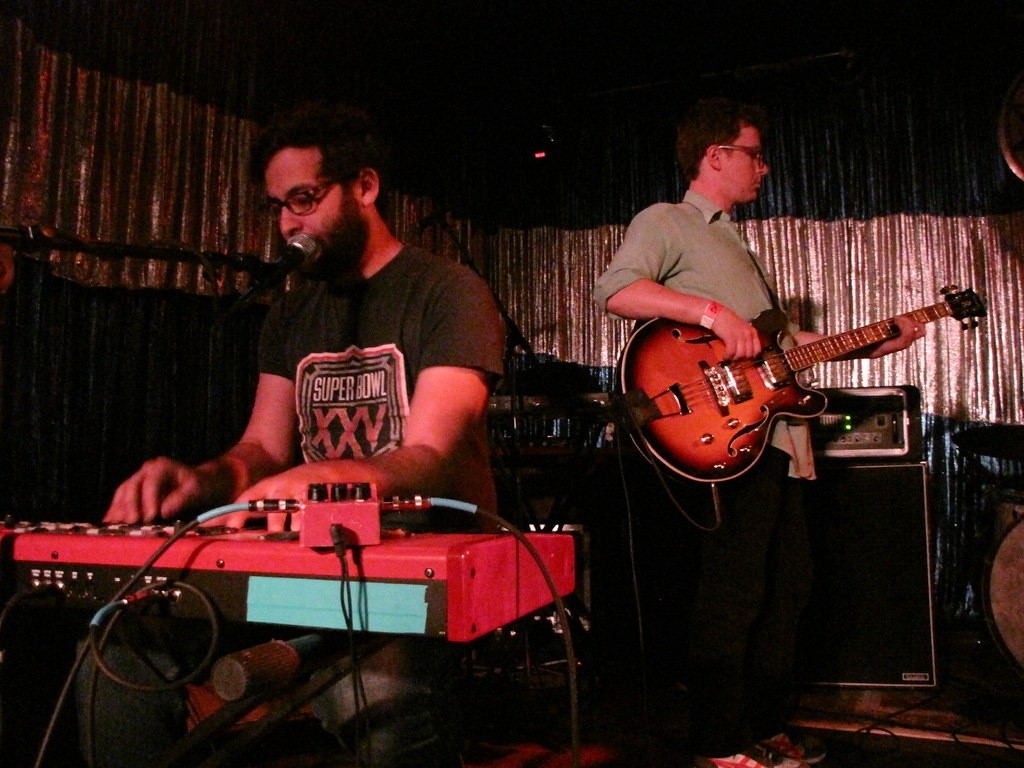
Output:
[787,460,936,689]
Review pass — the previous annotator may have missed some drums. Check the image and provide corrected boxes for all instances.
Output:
[980,508,1024,680]
[972,485,1024,572]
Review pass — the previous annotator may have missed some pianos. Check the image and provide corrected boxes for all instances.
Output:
[8,518,589,645]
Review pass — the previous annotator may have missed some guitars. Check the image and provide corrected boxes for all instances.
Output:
[608,282,991,489]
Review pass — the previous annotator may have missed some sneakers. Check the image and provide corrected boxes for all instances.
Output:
[694,744,809,768]
[757,733,826,764]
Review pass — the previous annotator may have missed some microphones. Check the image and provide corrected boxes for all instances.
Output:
[249,235,317,295]
[411,207,446,235]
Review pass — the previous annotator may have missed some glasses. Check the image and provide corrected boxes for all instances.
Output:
[260,175,340,220]
[715,146,772,168]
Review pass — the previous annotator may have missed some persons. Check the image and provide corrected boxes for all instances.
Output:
[594,100,925,768]
[78,98,505,768]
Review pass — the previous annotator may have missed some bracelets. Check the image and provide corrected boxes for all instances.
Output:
[700,301,723,329]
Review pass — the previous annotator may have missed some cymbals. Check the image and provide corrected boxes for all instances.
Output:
[950,424,1024,461]
